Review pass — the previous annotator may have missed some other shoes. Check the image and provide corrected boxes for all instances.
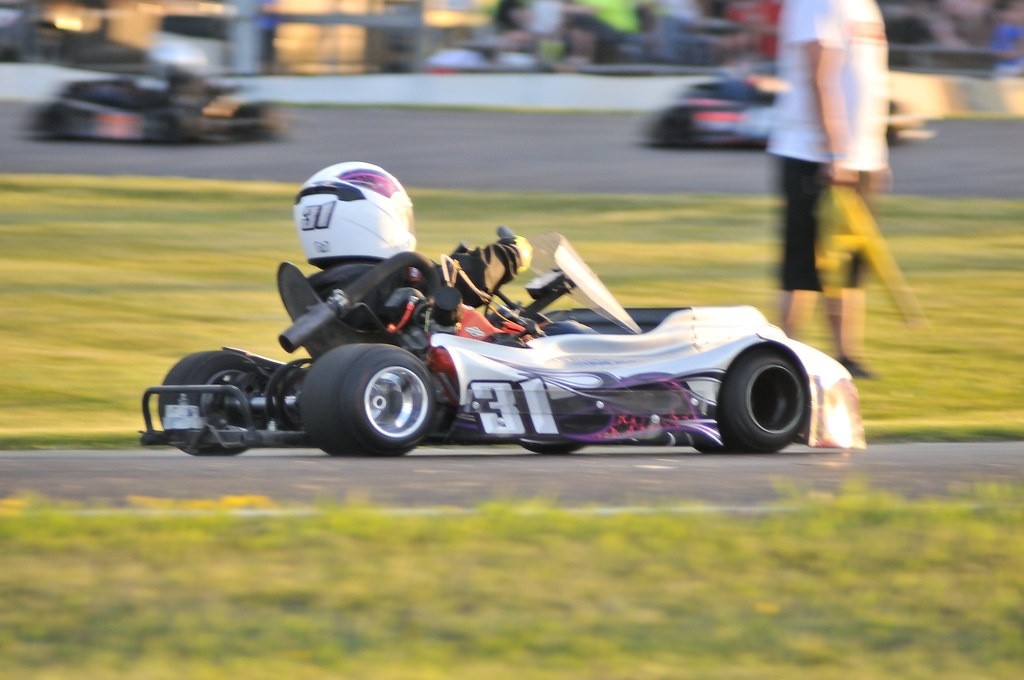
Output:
[839,354,881,379]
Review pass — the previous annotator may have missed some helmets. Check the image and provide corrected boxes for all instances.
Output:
[293,162,417,271]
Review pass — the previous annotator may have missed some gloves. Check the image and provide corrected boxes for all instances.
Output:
[496,224,533,274]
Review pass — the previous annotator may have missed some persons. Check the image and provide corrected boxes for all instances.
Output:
[0,1,1023,115]
[293,161,545,344]
[764,1,885,382]
